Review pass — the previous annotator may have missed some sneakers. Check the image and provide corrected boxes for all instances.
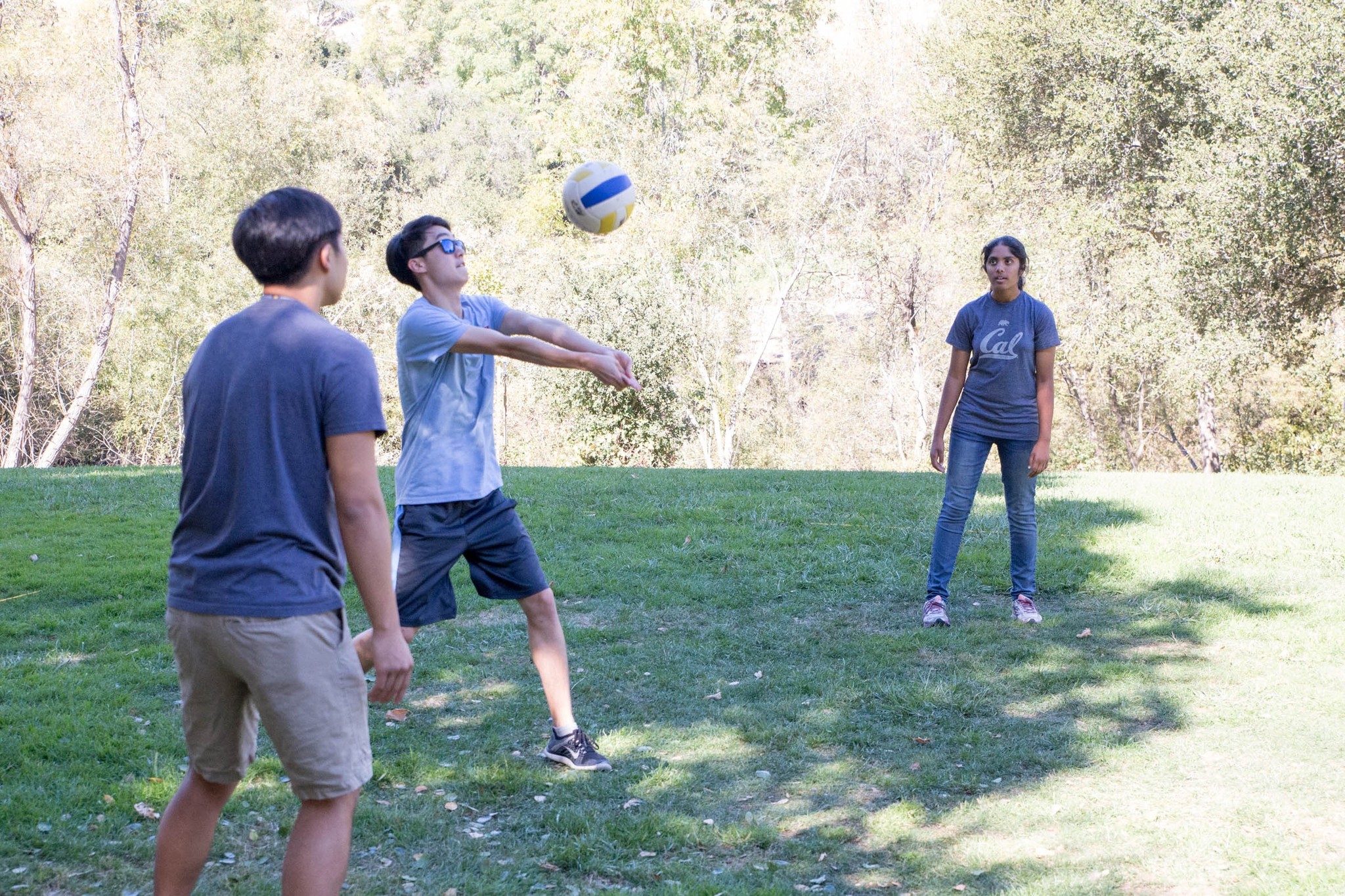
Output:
[922,595,951,628]
[543,727,613,772]
[1010,594,1043,624]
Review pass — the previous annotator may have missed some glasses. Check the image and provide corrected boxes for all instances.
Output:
[415,238,466,260]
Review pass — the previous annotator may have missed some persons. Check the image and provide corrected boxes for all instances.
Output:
[350,216,642,773]
[921,236,1060,627]
[151,186,416,896]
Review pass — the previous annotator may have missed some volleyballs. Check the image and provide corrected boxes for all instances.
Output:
[561,161,636,236]
